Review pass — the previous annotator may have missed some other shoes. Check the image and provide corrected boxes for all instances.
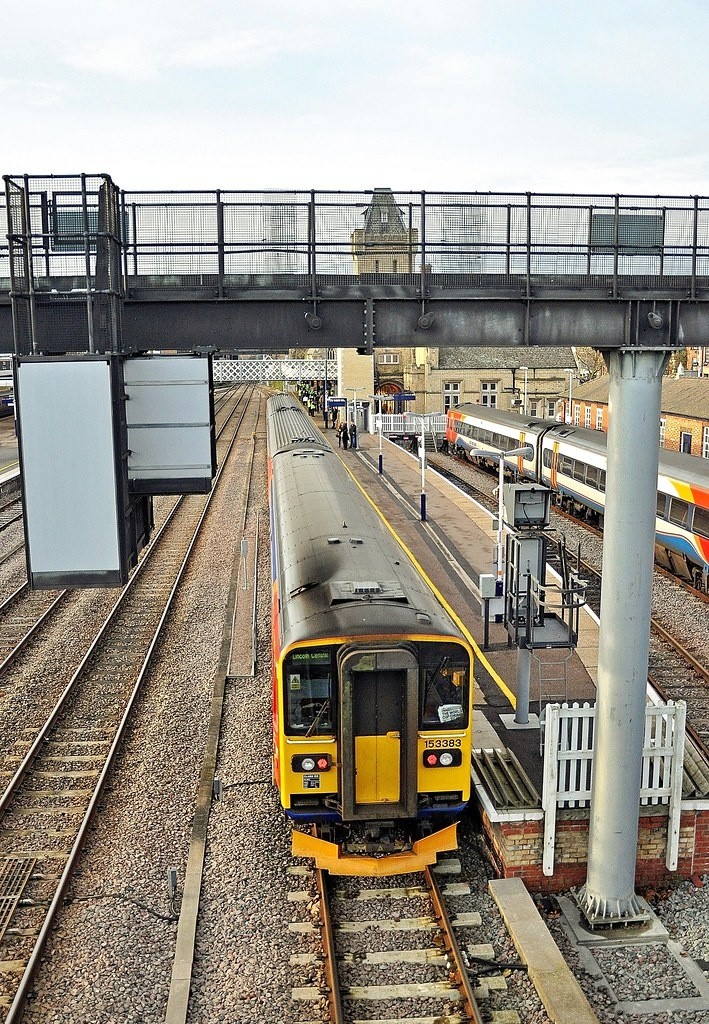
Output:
[312,415,315,417]
[349,446,352,448]
[343,448,347,450]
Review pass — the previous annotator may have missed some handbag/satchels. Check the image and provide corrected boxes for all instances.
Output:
[336,433,339,437]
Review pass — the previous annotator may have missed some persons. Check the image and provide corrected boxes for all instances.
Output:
[299,382,357,451]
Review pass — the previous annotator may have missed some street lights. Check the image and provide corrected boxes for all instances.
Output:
[519,366,529,415]
[563,369,574,416]
[469,448,534,624]
[344,386,366,426]
[403,411,442,523]
[367,394,393,475]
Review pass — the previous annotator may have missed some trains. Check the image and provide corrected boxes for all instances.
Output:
[446,401,709,598]
[265,389,473,878]
